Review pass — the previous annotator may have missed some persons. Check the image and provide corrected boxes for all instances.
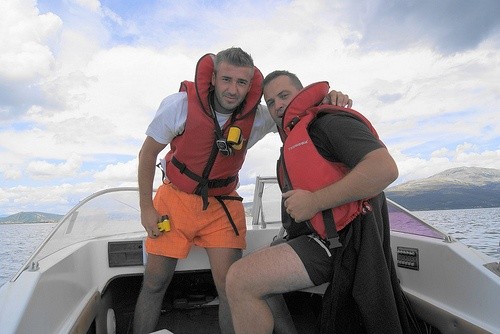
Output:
[130,47,354,334]
[225,71,401,334]
